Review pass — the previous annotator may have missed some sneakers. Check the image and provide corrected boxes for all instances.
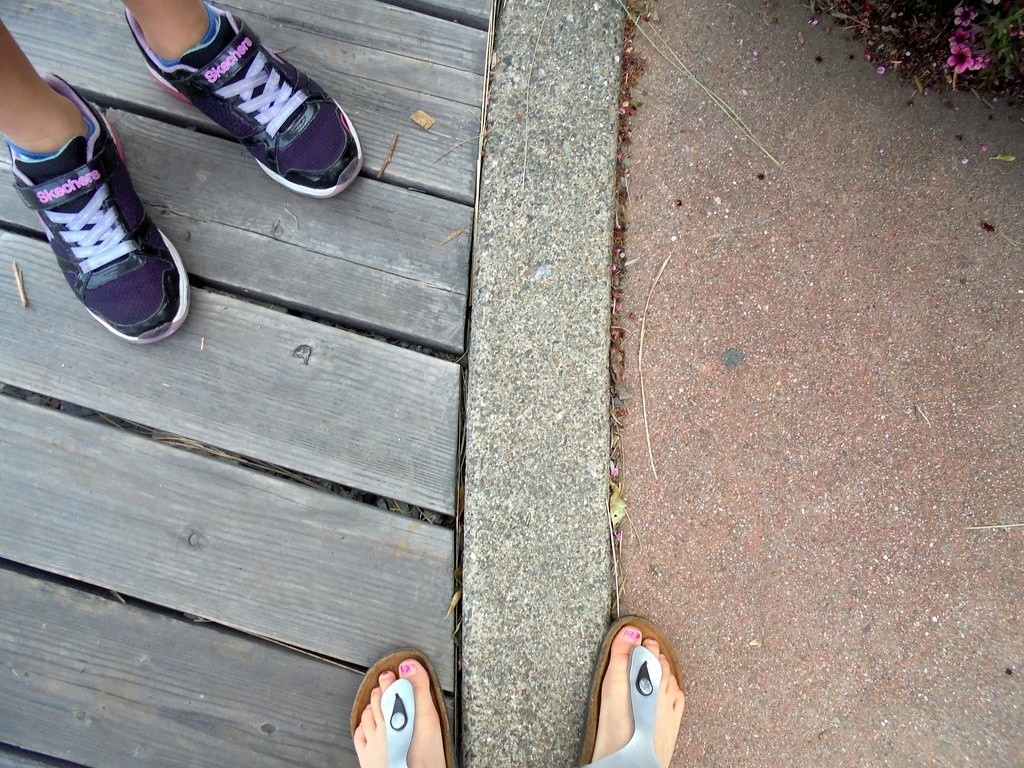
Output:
[124,0,363,200]
[0,70,191,344]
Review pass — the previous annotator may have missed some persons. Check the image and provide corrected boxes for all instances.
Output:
[351,615,689,768]
[1,1,364,345]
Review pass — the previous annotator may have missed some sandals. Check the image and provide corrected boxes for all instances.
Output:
[578,616,686,768]
[350,647,456,768]
